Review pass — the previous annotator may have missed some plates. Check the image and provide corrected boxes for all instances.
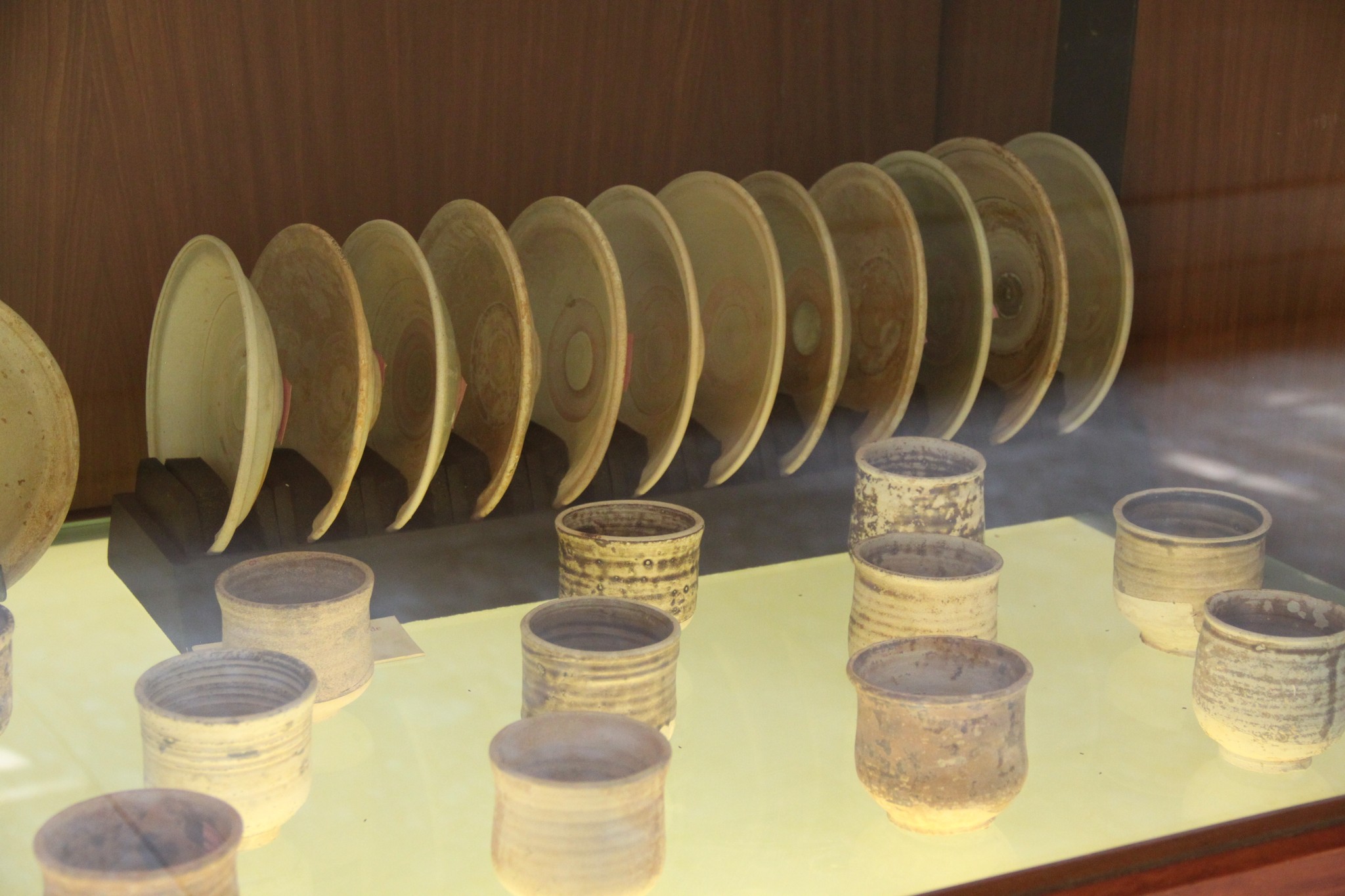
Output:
[508,196,628,509]
[586,184,705,499]
[655,170,786,488]
[1001,131,1134,435]
[341,219,462,534]
[926,137,1068,445]
[0,300,80,591]
[417,199,542,522]
[872,151,993,441]
[145,234,283,555]
[808,162,928,452]
[249,223,383,543]
[738,170,852,478]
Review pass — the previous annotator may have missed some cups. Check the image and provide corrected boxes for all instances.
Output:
[1113,487,1272,657]
[134,647,319,851]
[489,710,672,896]
[520,595,681,742]
[846,635,1033,835]
[32,789,244,896]
[848,532,1003,659]
[0,603,14,736]
[554,500,705,631]
[214,551,374,724]
[848,436,987,566]
[1192,588,1345,774]
